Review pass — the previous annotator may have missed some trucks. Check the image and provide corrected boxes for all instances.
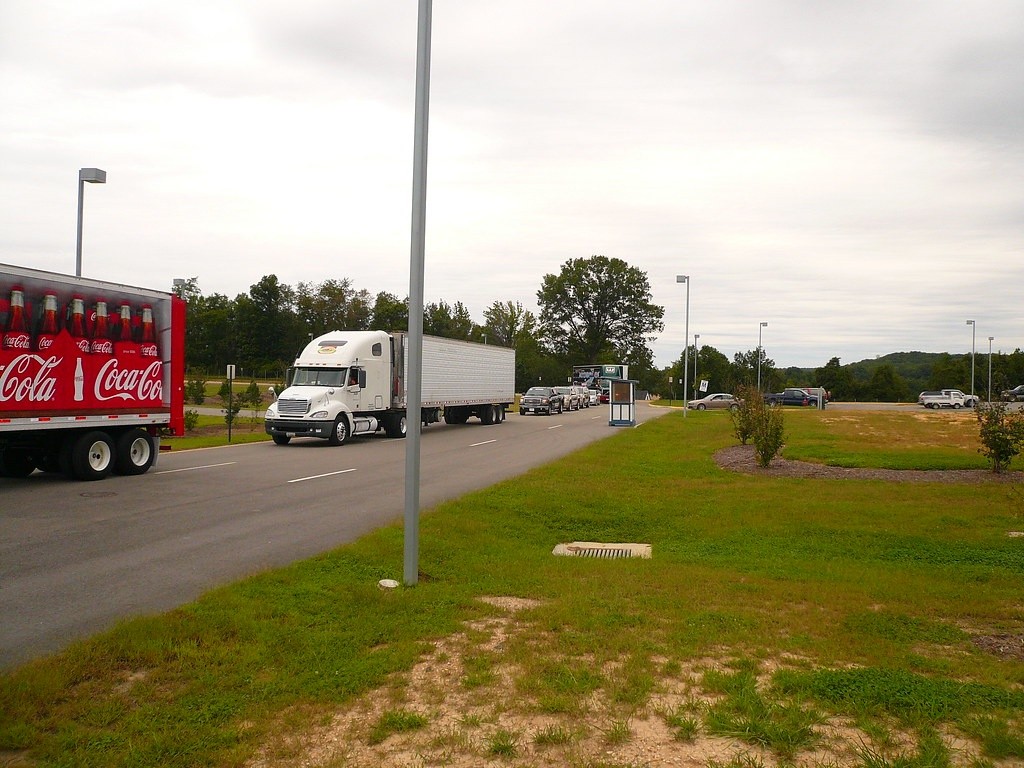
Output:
[0,262,187,480]
[919,388,981,407]
[924,391,965,410]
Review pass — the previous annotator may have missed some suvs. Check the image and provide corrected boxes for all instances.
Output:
[999,384,1024,401]
[801,387,831,404]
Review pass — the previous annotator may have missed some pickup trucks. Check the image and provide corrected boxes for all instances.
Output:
[762,388,819,407]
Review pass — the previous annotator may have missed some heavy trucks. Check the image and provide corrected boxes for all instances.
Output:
[264,329,516,446]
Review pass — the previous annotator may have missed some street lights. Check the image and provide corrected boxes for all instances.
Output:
[677,275,690,417]
[693,334,699,399]
[172,278,186,300]
[988,337,995,402]
[758,322,768,391]
[966,319,976,406]
[75,166,107,276]
[481,333,488,344]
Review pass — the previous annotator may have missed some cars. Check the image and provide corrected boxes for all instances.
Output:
[519,386,565,416]
[552,386,611,411]
[688,393,746,412]
